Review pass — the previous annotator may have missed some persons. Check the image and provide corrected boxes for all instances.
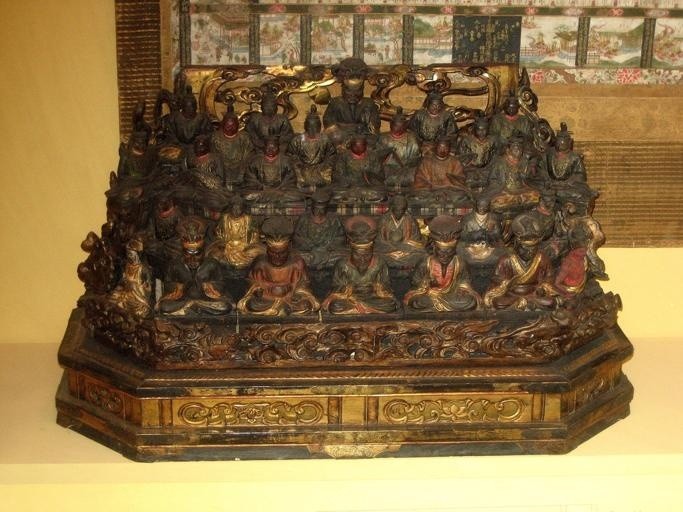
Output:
[77,46,610,316]
